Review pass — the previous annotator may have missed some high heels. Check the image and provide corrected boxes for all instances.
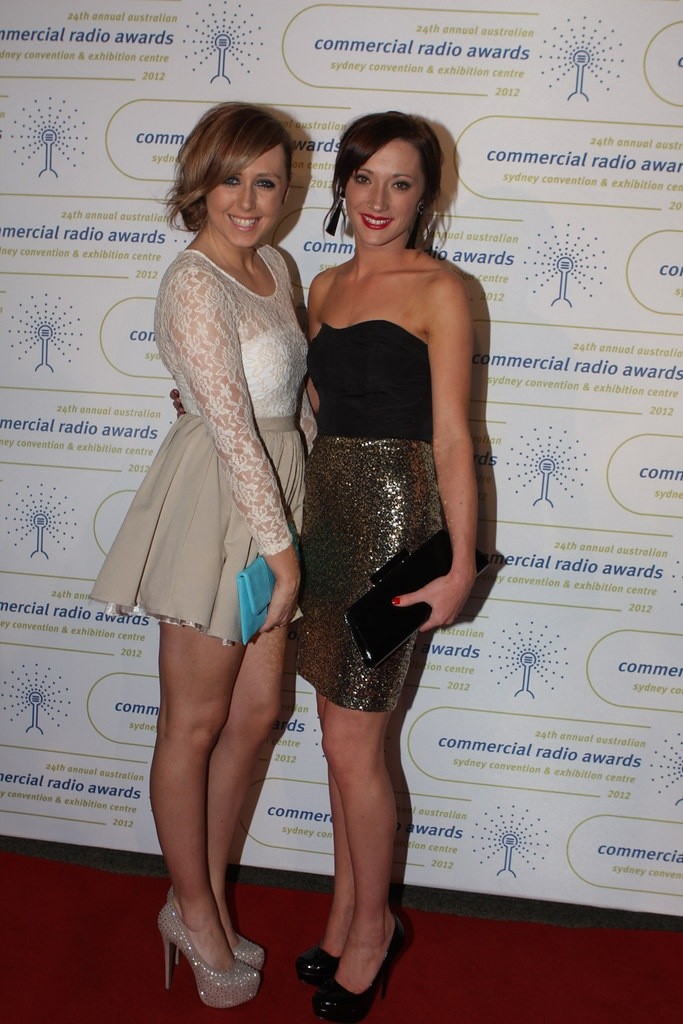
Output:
[168,886,266,972]
[156,896,260,1008]
[295,943,341,984]
[312,912,406,1023]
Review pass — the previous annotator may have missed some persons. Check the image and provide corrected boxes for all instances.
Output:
[89,101,310,1006]
[171,110,478,1024]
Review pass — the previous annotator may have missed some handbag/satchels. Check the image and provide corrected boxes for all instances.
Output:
[345,530,493,668]
[236,522,301,645]
